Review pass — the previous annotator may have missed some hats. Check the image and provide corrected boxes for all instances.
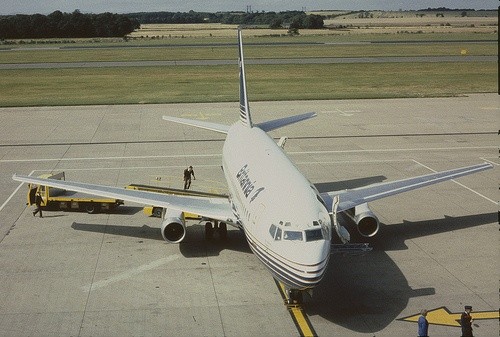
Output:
[464,306,472,309]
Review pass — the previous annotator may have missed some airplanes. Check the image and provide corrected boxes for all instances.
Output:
[10,25,494,313]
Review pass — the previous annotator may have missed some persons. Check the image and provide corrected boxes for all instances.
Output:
[417,308,429,337]
[184,166,195,189]
[32,192,45,218]
[461,305,474,337]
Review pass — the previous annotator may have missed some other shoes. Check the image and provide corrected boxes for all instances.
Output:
[39,217,44,218]
[33,212,35,216]
